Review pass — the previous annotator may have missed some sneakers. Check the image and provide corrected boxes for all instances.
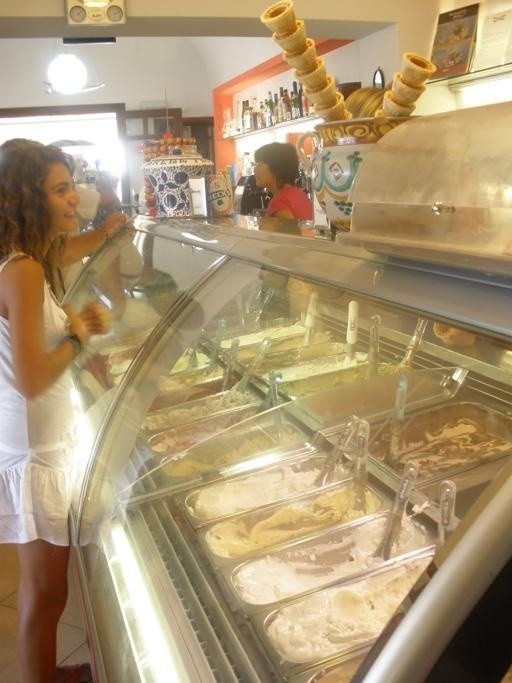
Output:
[56,663,93,683]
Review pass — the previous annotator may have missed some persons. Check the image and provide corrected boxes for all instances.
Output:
[253,143,316,320]
[92,172,122,230]
[0,139,127,303]
[0,140,115,683]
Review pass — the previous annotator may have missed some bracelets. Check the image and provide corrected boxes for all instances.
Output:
[61,334,82,359]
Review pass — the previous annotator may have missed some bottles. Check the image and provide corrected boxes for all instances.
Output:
[238,151,252,176]
[223,79,307,137]
